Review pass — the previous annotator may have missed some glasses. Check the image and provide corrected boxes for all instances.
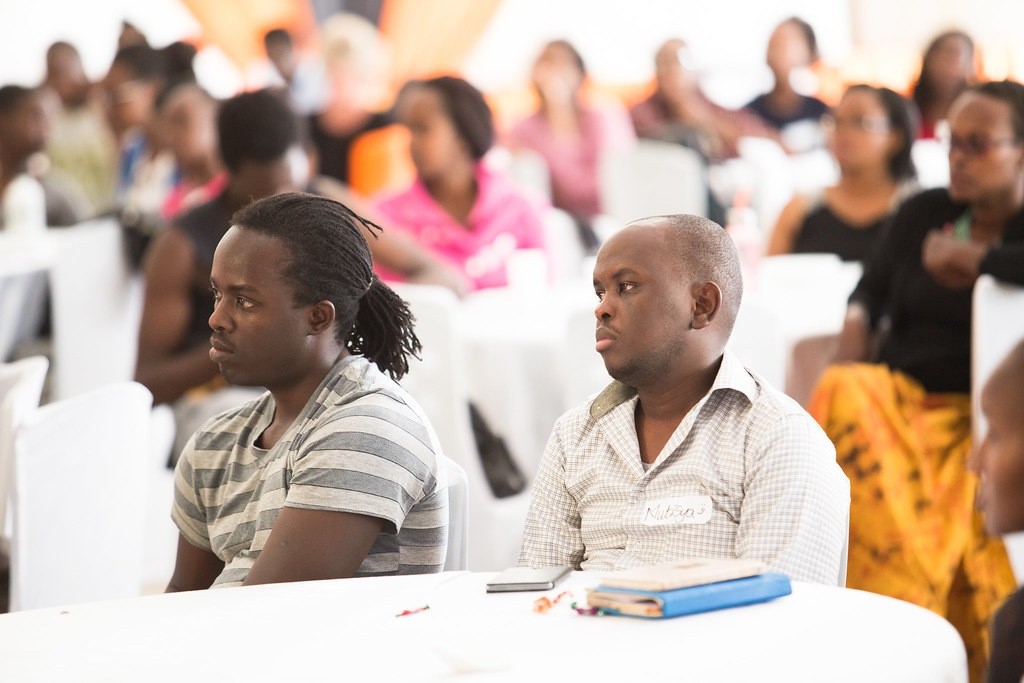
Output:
[941,127,1022,154]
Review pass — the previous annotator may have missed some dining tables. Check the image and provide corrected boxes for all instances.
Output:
[461,139,965,565]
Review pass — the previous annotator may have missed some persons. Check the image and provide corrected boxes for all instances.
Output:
[763,78,1024,683]
[511,16,991,278]
[516,213,854,588]
[135,91,451,594]
[0,13,546,291]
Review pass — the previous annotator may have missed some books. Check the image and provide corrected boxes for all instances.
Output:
[485,565,572,593]
[586,557,793,620]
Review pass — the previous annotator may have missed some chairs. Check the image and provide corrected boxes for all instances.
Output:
[0,145,705,611]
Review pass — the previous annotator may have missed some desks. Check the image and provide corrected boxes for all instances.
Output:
[0,568,970,683]
